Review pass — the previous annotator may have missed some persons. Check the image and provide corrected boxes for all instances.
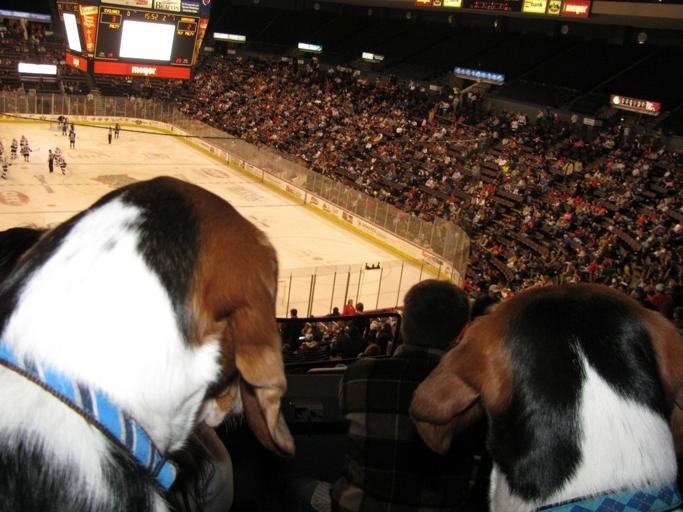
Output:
[0,226,49,284]
[282,183,682,510]
[1,16,683,183]
[166,420,233,511]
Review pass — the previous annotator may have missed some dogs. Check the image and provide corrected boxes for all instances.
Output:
[408,281,683,511]
[0,174,297,511]
